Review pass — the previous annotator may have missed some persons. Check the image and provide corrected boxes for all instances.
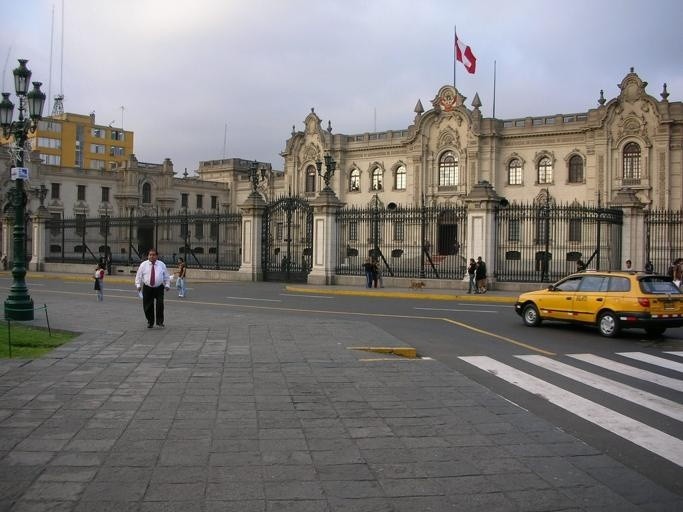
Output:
[667,257,683,286]
[374,259,385,289]
[360,255,378,289]
[92,261,108,302]
[174,257,188,298]
[476,256,487,295]
[624,258,634,271]
[135,248,172,330]
[465,257,476,294]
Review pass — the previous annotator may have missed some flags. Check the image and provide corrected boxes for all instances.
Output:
[454,32,479,75]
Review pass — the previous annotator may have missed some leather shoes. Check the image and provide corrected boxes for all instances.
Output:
[157,324,164,328]
[147,323,154,328]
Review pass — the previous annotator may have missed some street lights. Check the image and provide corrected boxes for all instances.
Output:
[245,160,266,194]
[314,153,338,188]
[0,59,45,321]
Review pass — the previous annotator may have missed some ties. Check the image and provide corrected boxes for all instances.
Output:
[151,261,155,286]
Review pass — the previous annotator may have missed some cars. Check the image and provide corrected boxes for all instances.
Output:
[513,271,683,338]
